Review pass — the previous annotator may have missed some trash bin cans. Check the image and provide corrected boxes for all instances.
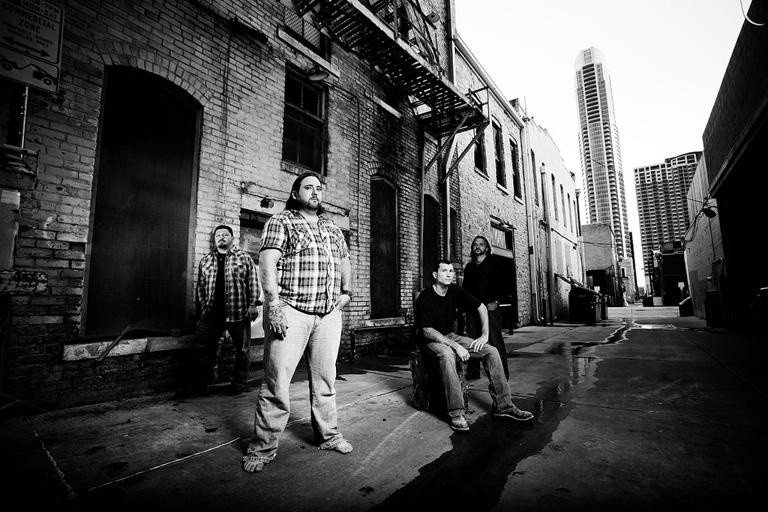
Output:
[678,296,694,318]
[568,287,608,323]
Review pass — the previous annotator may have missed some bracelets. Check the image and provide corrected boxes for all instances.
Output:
[495,301,500,305]
[343,290,353,300]
[267,300,280,306]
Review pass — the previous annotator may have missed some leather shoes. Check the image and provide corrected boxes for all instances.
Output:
[494,407,534,420]
[448,416,470,431]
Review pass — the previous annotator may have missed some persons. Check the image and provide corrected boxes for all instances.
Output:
[196,225,261,394]
[462,236,516,382]
[242,172,353,473]
[414,260,534,432]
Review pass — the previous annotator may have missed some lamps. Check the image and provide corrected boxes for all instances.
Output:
[426,0,440,23]
[702,204,719,219]
[307,64,330,82]
